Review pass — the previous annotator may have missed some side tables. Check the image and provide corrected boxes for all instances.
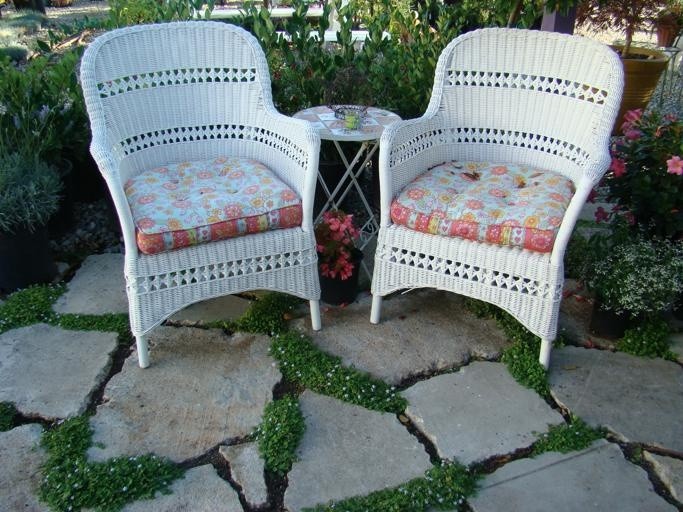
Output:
[293,104,404,284]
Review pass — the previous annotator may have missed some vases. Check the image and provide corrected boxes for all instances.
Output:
[319,248,363,305]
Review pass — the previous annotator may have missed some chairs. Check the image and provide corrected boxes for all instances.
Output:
[368,27,626,370]
[79,22,324,368]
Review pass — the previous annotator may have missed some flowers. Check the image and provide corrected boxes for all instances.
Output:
[314,210,361,280]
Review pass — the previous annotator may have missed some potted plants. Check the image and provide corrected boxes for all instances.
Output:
[569,0,674,136]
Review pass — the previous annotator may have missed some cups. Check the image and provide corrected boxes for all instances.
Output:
[344,110,361,131]
